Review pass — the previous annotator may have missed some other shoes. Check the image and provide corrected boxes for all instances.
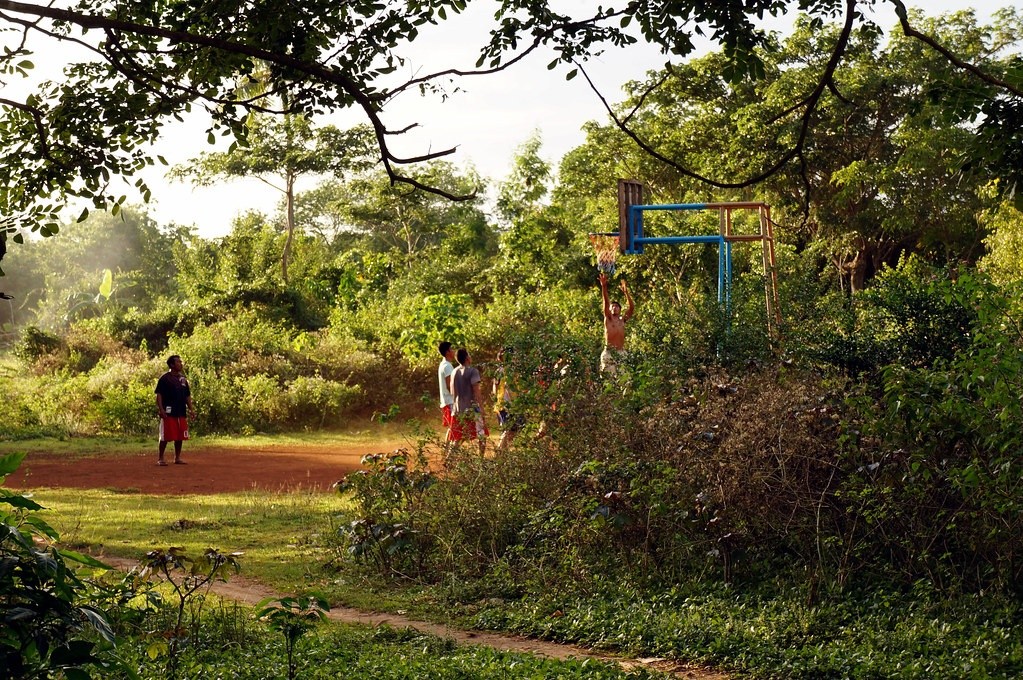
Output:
[157,459,168,466]
[173,460,189,465]
[442,461,454,469]
[443,443,450,451]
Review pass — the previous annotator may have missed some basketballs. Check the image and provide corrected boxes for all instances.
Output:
[598,250,615,265]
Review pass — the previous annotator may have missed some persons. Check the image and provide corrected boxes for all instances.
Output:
[154,355,195,466]
[597,273,634,379]
[438,342,592,467]
